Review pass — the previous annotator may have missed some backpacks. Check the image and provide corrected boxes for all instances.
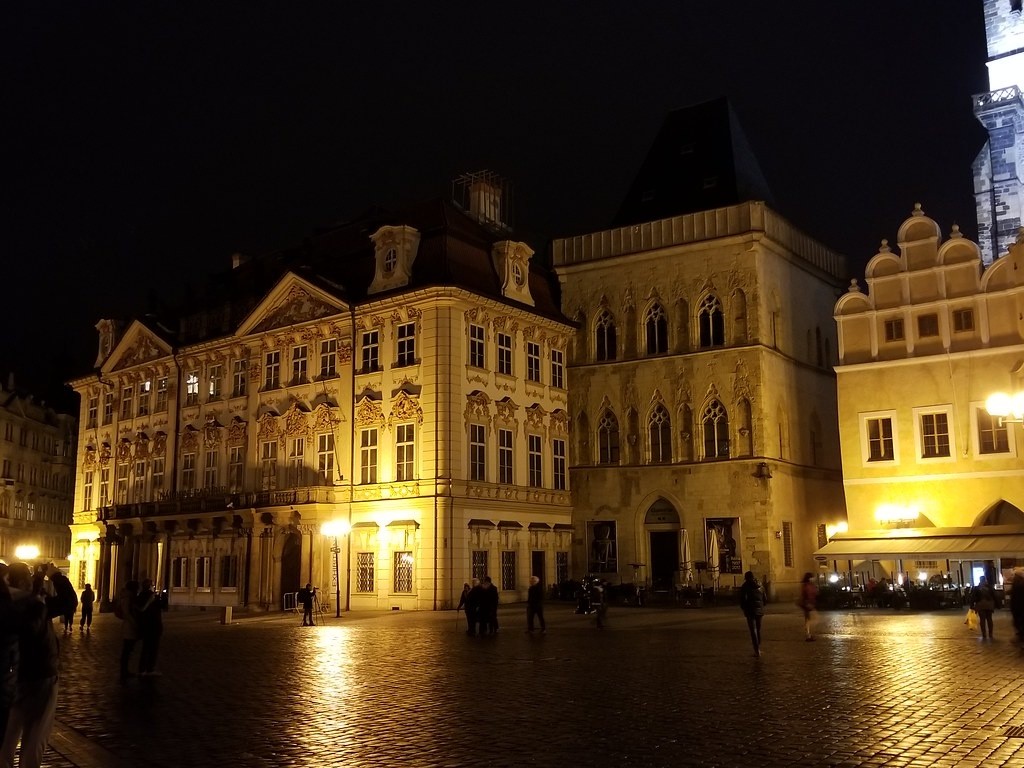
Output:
[298,588,307,602]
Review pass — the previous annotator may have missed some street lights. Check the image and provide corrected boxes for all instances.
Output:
[321,517,348,618]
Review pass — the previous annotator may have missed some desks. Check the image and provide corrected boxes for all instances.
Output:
[815,571,969,610]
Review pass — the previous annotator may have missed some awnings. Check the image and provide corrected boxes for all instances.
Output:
[813,523,1024,562]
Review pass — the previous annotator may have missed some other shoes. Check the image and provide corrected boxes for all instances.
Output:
[80,626,83,631]
[87,628,90,631]
[138,671,162,676]
[806,635,816,641]
[309,623,316,626]
[118,671,135,677]
[69,631,72,633]
[753,650,763,657]
[64,630,68,633]
[303,623,308,626]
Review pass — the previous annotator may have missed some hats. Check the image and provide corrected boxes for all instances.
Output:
[980,576,985,580]
[484,576,491,583]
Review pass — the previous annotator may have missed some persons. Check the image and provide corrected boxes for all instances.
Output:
[456,575,499,642]
[736,571,766,657]
[798,572,820,641]
[867,576,888,606]
[524,576,549,635]
[970,575,999,643]
[1,561,169,767]
[302,583,316,626]
